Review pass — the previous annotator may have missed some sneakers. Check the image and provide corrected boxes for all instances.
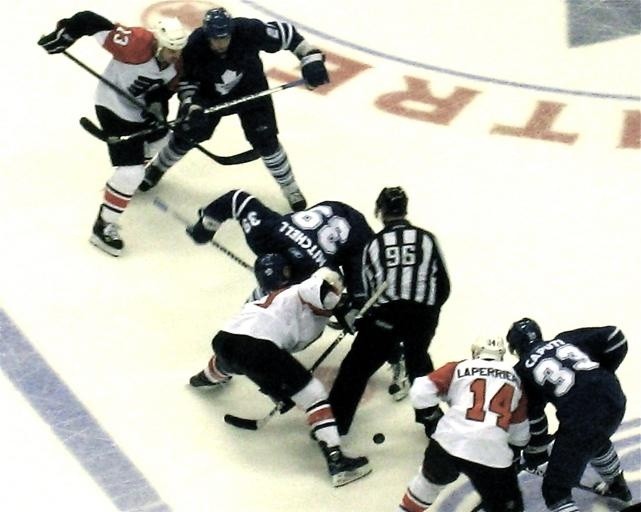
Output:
[593,472,632,501]
[322,445,369,476]
[92,216,124,250]
[138,165,165,191]
[388,358,410,402]
[283,184,306,212]
[189,371,232,387]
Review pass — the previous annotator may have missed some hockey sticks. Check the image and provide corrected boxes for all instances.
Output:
[224,281,388,430]
[153,198,343,330]
[79,80,304,144]
[40,34,260,166]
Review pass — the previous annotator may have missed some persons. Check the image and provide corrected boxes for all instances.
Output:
[138,8,331,213]
[191,190,412,395]
[37,11,189,251]
[507,318,632,512]
[190,267,369,476]
[310,188,450,442]
[395,334,532,512]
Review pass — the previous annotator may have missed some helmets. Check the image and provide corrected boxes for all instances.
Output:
[313,266,344,294]
[375,187,406,222]
[506,318,543,357]
[472,335,504,360]
[156,17,187,49]
[254,251,291,290]
[204,9,233,37]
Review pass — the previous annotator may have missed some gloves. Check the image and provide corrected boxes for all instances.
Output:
[143,104,168,143]
[180,97,205,132]
[37,28,76,53]
[523,451,549,470]
[301,51,329,90]
[187,208,222,243]
[414,404,444,437]
